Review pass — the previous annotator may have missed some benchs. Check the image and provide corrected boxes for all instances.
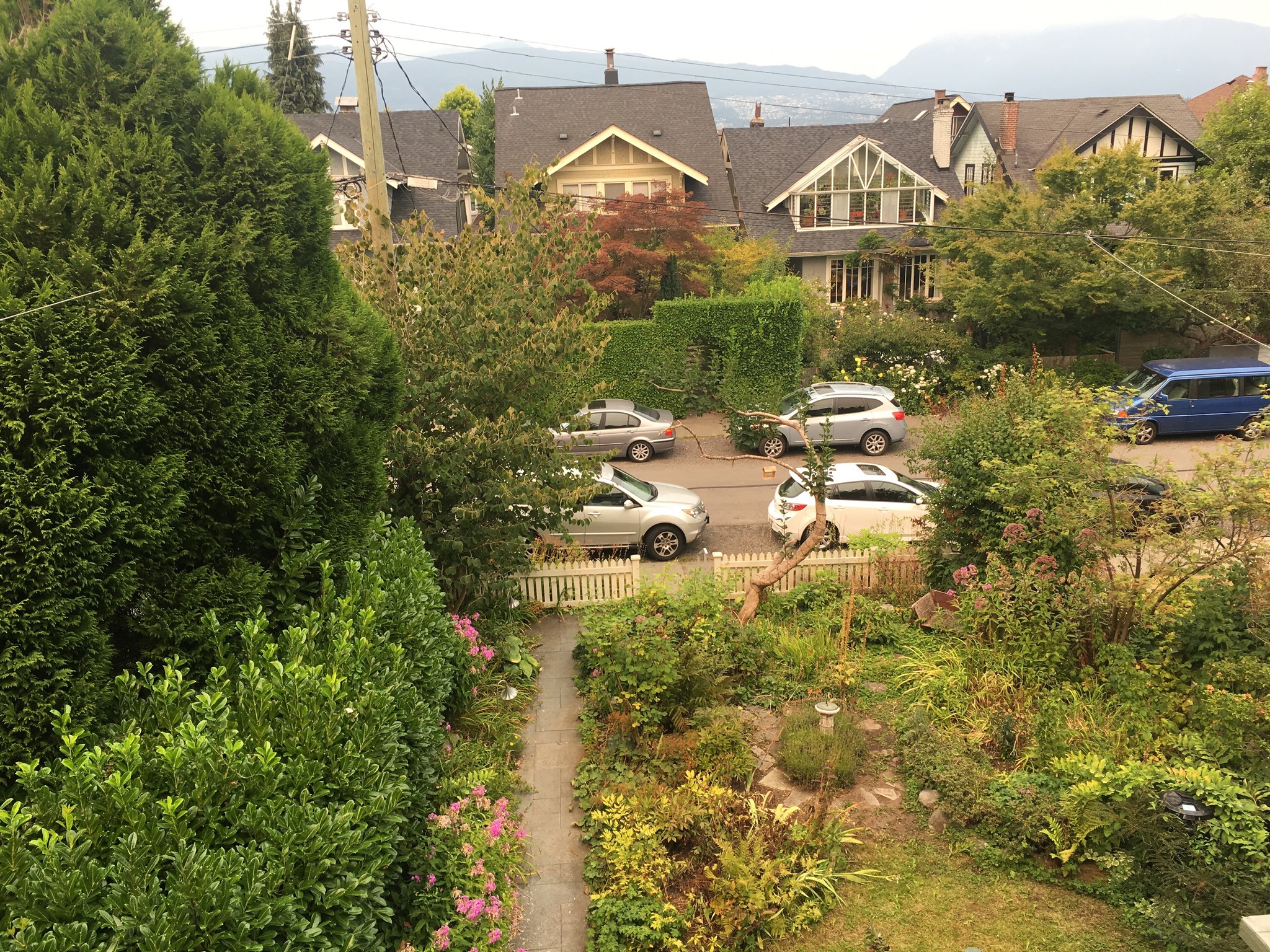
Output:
[1245,383,1264,396]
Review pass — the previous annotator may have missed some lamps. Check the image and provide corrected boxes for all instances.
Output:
[510,106,519,116]
[514,88,523,99]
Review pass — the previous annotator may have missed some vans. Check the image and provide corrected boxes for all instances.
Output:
[1097,357,1270,446]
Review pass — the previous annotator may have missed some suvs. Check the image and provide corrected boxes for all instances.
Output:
[496,461,710,562]
[731,381,908,458]
[767,462,945,552]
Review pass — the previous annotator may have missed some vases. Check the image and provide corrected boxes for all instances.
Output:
[849,216,855,222]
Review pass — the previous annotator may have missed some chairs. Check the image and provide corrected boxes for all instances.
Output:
[1160,382,1176,394]
[1168,386,1188,399]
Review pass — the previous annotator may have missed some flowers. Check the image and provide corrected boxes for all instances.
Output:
[849,210,864,217]
[886,172,895,184]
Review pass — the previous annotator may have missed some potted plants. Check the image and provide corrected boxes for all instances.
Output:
[800,172,927,224]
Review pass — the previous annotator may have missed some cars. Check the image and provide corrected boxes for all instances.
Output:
[539,399,676,463]
